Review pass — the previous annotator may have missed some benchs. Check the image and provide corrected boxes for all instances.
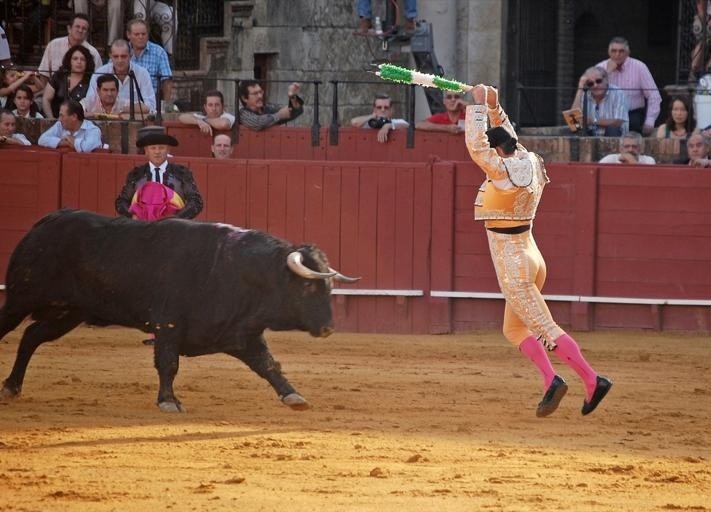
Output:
[516,84,711,167]
[15,117,144,154]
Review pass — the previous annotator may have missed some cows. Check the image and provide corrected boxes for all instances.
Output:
[0,207,363,414]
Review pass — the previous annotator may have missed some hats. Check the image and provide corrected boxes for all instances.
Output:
[135,133,179,147]
[485,125,511,148]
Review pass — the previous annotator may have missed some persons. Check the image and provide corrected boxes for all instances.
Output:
[0,12,173,152]
[114,124,206,347]
[355,0,418,38]
[239,81,304,132]
[210,133,233,159]
[346,95,410,145]
[647,92,702,138]
[678,132,711,167]
[593,36,662,137]
[569,64,632,139]
[416,87,472,135]
[596,129,659,166]
[462,81,615,426]
[178,90,235,136]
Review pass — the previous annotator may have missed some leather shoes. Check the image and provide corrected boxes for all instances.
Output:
[582,375,613,415]
[536,374,568,417]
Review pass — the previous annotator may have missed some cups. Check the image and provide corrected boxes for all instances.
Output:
[161,100,167,114]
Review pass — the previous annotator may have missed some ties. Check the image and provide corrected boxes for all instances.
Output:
[154,167,160,183]
